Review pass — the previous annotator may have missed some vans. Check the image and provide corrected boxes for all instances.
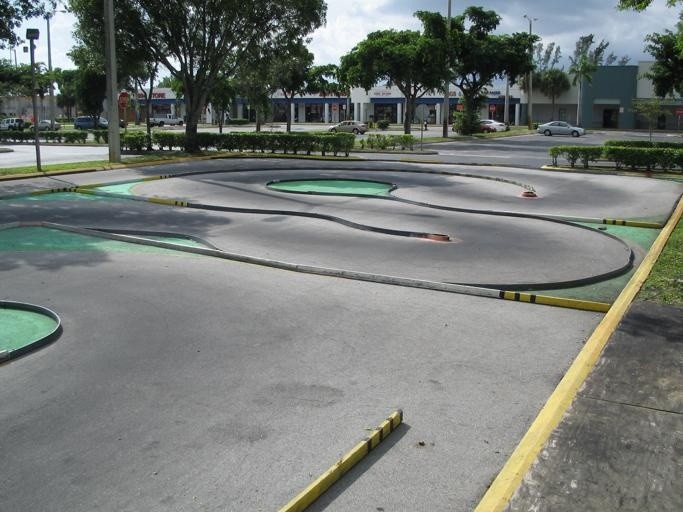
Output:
[74,115,107,130]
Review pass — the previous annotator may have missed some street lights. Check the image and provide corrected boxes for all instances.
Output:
[522,14,537,128]
[25,28,41,171]
[45,9,65,133]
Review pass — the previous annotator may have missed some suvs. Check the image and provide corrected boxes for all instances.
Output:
[0,118,24,133]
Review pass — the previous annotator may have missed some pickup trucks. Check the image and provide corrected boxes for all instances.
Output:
[145,113,183,129]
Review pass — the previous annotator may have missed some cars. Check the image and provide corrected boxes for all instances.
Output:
[535,121,585,137]
[29,119,61,132]
[452,119,507,135]
[328,121,367,135]
[119,119,128,127]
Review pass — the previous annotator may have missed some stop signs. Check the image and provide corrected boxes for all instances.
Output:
[118,92,131,110]
[489,105,495,112]
